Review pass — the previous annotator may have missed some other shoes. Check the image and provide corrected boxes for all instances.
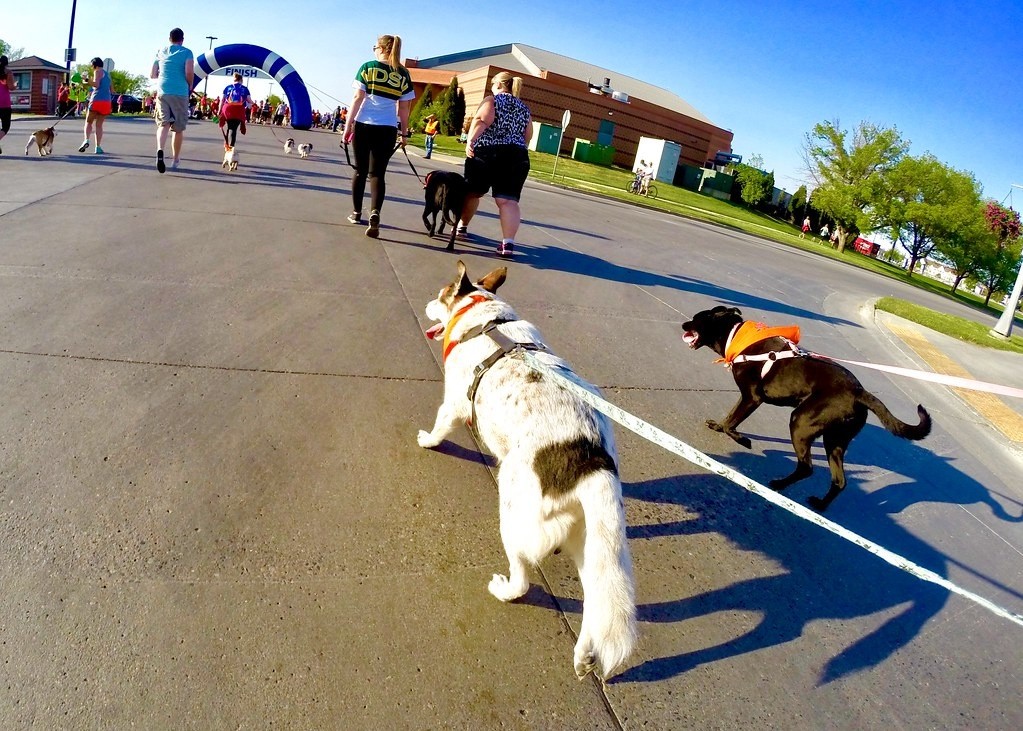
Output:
[156,150,168,174]
[423,156,430,159]
[348,211,361,224]
[79,140,90,152]
[366,214,380,238]
[95,147,104,154]
[172,157,181,171]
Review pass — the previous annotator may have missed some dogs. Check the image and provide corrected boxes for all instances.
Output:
[283,138,313,158]
[681,305,932,515]
[25,127,57,157]
[222,143,239,171]
[421,170,468,252]
[417,260,637,685]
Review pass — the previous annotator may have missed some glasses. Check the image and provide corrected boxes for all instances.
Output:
[373,45,381,52]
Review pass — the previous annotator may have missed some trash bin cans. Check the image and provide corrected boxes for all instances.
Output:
[572,137,615,167]
[679,162,704,190]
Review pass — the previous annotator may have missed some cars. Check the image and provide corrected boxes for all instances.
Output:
[111,94,143,114]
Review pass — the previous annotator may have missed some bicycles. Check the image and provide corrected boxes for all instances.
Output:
[626,172,658,199]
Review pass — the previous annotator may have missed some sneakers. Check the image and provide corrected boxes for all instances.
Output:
[496,243,514,255]
[450,225,467,237]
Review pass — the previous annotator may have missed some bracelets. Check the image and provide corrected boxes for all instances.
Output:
[402,134,408,138]
[85,78,89,82]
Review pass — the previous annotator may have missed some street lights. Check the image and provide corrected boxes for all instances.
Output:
[204,36,217,95]
[269,83,274,95]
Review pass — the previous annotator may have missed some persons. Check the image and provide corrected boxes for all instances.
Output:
[246,99,292,126]
[189,94,198,117]
[150,28,193,173]
[57,82,89,118]
[341,35,415,237]
[312,106,348,132]
[423,113,440,160]
[200,94,211,114]
[218,72,255,147]
[117,93,123,114]
[456,71,533,258]
[799,217,812,239]
[142,93,156,114]
[194,107,203,120]
[213,96,220,114]
[630,160,654,197]
[78,57,115,154]
[829,228,838,249]
[0,55,16,155]
[818,224,830,245]
